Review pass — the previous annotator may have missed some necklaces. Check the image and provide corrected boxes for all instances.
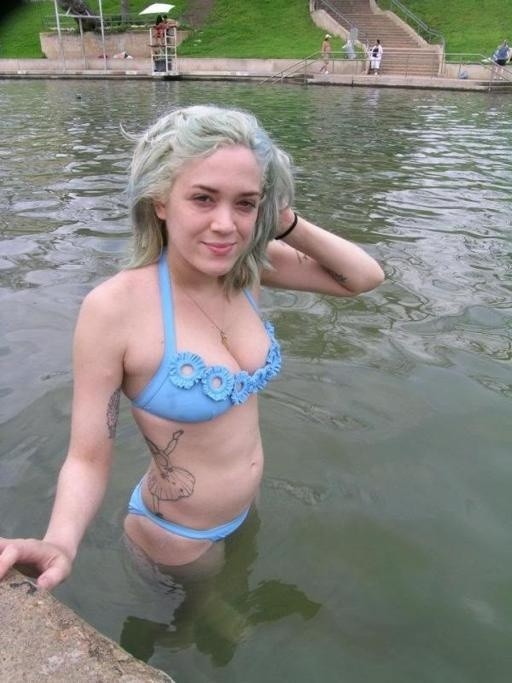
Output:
[179,280,230,349]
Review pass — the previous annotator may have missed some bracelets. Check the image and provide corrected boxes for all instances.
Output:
[274,210,297,239]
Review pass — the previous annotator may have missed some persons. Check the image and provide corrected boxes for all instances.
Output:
[0,100,387,658]
[151,15,170,47]
[371,38,383,74]
[319,33,332,74]
[493,38,510,79]
